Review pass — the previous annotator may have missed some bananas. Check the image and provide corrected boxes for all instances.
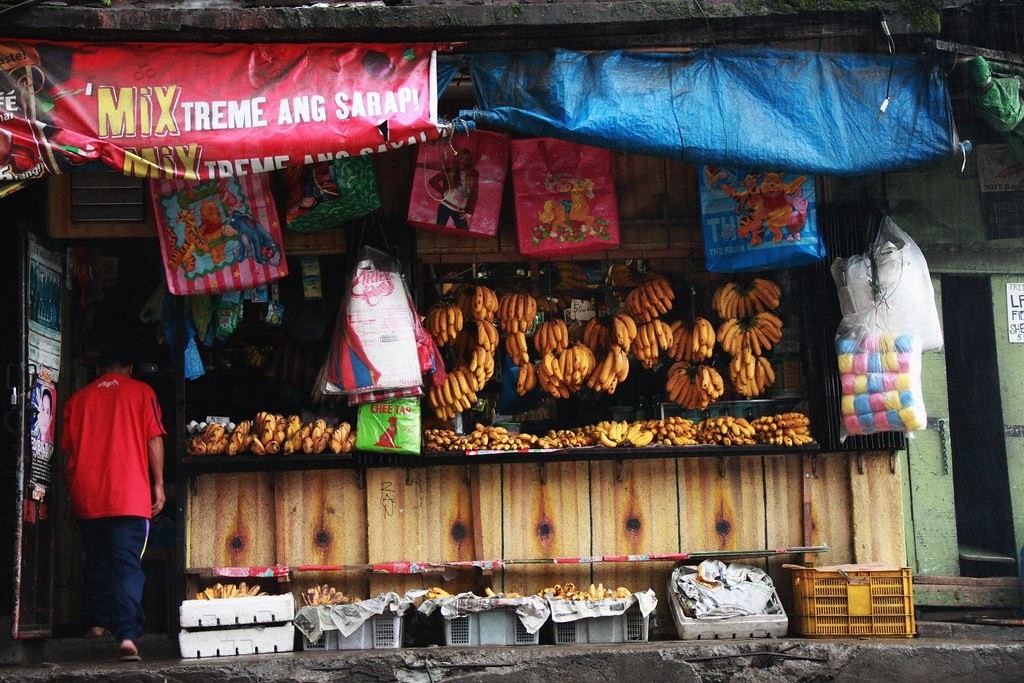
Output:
[301,584,361,606]
[195,582,266,599]
[186,412,355,454]
[426,277,811,450]
[426,582,631,600]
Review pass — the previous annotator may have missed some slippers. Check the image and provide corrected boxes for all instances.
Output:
[83,625,113,640]
[115,654,143,662]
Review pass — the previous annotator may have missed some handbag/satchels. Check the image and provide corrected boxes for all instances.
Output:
[405,129,511,239]
[698,164,827,275]
[150,172,289,296]
[509,137,619,258]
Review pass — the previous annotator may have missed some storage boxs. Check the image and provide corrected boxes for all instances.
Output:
[179,592,296,628]
[294,604,405,651]
[551,600,649,645]
[791,569,917,639]
[438,597,540,646]
[178,622,296,659]
[666,580,789,640]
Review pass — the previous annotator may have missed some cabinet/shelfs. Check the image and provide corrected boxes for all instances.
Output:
[81,270,185,640]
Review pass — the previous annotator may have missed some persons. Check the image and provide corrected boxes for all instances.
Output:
[430,148,480,231]
[35,389,54,446]
[62,346,165,661]
